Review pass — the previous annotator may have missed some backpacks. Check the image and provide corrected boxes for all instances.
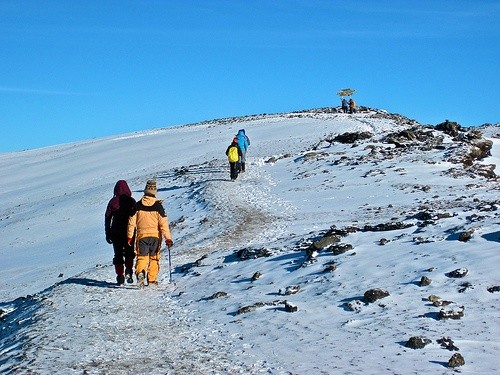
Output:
[228,147,238,163]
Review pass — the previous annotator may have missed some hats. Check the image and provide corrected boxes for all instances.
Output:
[233,138,238,142]
[144,179,157,195]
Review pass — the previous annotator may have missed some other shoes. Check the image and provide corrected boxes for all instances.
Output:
[148,281,158,286]
[118,280,124,284]
[125,274,133,283]
[137,273,144,290]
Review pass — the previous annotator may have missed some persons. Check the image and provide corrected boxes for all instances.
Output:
[236,129,250,173]
[226,138,242,182]
[349,99,354,113]
[105,180,137,286]
[342,99,350,113]
[127,181,174,288]
